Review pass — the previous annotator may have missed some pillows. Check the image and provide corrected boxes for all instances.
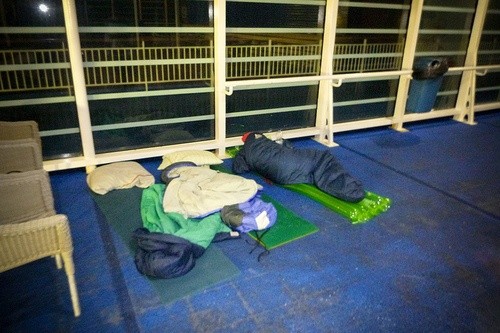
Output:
[86,161,155,195]
[157,150,224,170]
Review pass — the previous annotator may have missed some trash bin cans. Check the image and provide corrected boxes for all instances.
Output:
[406,57,450,114]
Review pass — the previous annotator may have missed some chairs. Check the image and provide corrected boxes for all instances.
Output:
[0,121,81,317]
